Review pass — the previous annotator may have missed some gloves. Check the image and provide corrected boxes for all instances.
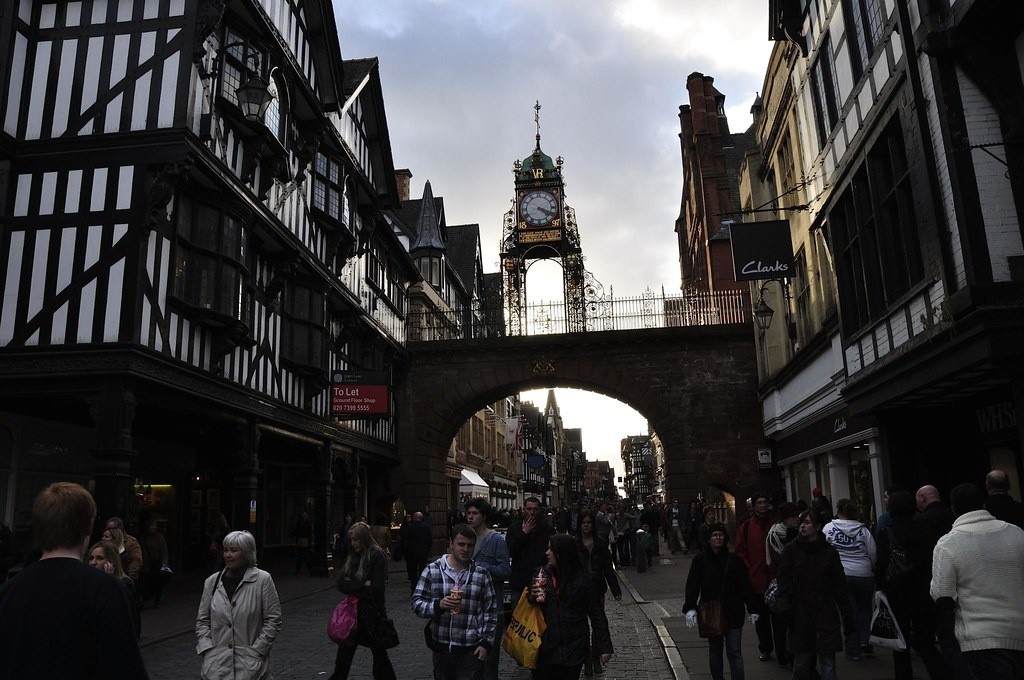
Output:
[747,614,760,625]
[685,609,697,630]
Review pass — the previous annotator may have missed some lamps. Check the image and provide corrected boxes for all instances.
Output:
[200,42,276,141]
[752,279,797,341]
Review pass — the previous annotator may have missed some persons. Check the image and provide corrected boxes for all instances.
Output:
[195,530,282,680]
[289,469,1024,680]
[0,512,231,642]
[0,481,152,680]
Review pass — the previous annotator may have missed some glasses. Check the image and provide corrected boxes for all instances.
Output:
[786,511,801,518]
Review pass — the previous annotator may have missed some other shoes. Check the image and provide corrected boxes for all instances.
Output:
[684,549,689,555]
[758,651,770,662]
[860,642,874,655]
[777,649,790,666]
[405,578,410,582]
[847,655,861,661]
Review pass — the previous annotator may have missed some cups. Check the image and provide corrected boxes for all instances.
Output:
[450,590,463,614]
[534,578,547,602]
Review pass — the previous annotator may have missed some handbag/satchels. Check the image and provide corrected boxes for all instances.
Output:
[697,599,729,638]
[763,578,792,614]
[159,565,175,576]
[360,614,400,651]
[883,526,919,590]
[393,542,402,562]
[297,537,309,547]
[502,586,547,670]
[208,535,218,554]
[608,526,625,544]
[328,594,359,644]
[868,590,907,653]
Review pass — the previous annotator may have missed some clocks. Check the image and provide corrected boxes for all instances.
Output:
[519,190,559,227]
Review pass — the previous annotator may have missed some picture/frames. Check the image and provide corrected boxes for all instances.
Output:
[189,488,221,547]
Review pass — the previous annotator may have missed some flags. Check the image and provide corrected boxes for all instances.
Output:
[503,418,524,448]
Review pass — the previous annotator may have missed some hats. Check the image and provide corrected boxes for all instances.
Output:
[813,488,822,496]
[751,492,769,501]
[776,501,797,518]
